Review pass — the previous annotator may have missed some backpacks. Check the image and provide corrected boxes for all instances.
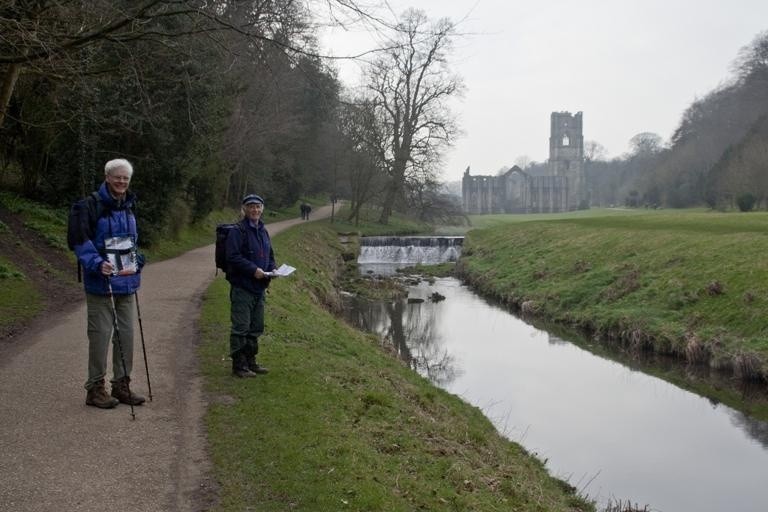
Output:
[66,192,103,251]
[215,221,250,271]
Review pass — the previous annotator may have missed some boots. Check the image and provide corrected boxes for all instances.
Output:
[85,380,119,408]
[109,375,146,405]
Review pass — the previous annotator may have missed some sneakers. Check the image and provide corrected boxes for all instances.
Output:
[231,367,256,377]
[250,366,269,374]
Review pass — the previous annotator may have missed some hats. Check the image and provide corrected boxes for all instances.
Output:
[242,194,263,205]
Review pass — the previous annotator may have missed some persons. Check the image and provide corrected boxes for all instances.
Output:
[306,202,311,221]
[224,194,278,377]
[66,157,146,409]
[301,201,306,220]
